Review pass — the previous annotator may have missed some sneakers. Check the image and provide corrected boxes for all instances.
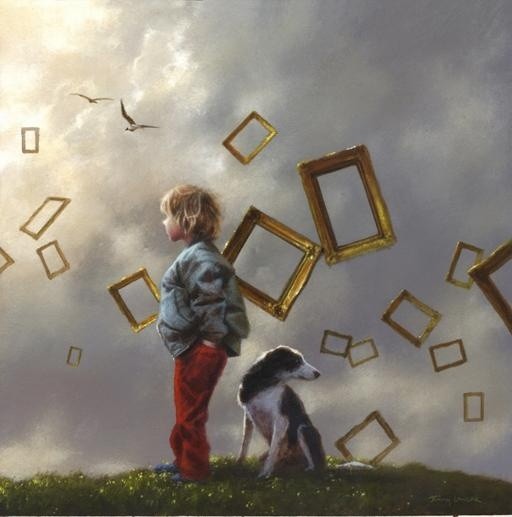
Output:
[155,464,178,473]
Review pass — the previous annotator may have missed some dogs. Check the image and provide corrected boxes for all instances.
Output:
[233,345,381,489]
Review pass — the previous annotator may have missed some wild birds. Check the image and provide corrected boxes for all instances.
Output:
[69,93,114,104]
[120,99,159,132]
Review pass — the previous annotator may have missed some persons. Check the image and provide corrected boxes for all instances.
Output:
[152,183,250,486]
